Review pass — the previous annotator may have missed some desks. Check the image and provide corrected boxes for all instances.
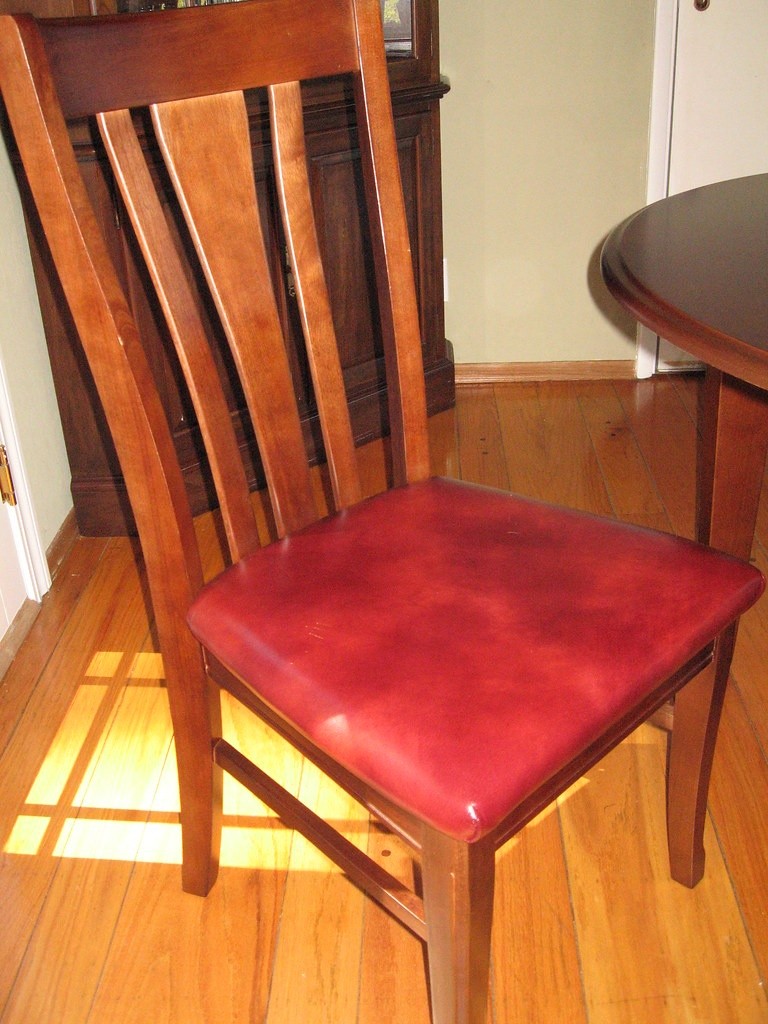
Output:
[586,173,768,889]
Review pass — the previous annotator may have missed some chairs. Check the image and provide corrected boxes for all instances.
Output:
[0,0,768,1024]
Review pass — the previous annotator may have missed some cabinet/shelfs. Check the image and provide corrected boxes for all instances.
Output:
[17,0,456,540]
